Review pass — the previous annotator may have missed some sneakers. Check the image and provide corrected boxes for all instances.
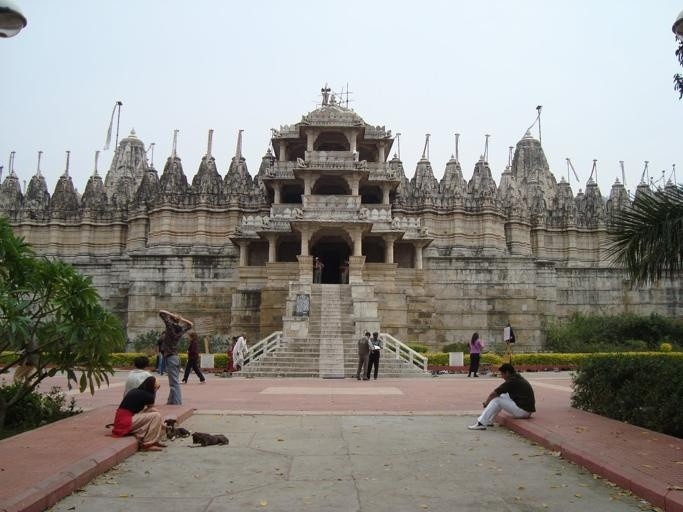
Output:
[138,441,168,452]
[467,421,495,430]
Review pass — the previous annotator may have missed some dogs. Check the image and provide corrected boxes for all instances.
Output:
[164,419,190,441]
[187,432,229,448]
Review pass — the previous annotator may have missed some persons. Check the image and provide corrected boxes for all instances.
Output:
[113,376,168,452]
[312,256,325,284]
[155,331,167,375]
[177,330,206,385]
[466,362,536,431]
[120,354,161,413]
[157,307,194,405]
[232,332,249,371]
[367,332,383,380]
[338,260,349,283]
[466,331,485,379]
[354,331,373,380]
